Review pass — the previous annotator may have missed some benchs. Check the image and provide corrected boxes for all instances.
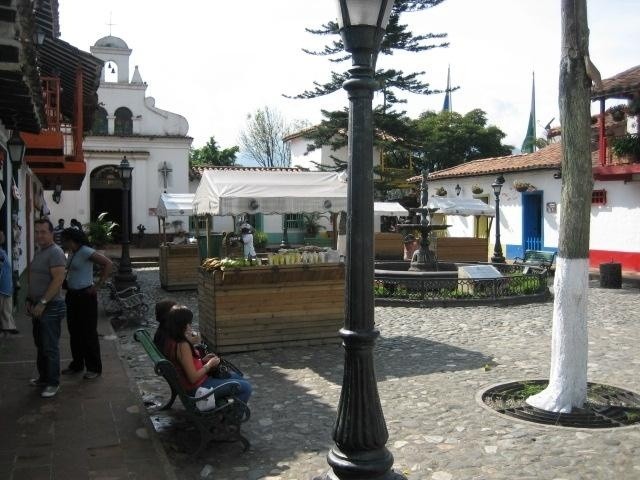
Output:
[510,247,557,277]
[106,281,150,328]
[130,329,253,463]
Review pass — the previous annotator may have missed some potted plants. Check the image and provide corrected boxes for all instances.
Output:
[612,132,640,165]
[513,178,536,193]
[608,103,626,121]
[435,185,448,197]
[471,184,484,194]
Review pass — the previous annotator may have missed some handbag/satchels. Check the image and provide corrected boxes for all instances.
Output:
[61,279,68,290]
[197,345,230,379]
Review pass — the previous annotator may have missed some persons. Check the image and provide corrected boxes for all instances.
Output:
[162,304,252,419]
[0,231,20,335]
[70,218,77,237]
[25,219,65,397]
[153,300,230,359]
[53,219,64,249]
[60,228,113,380]
[78,222,82,231]
[239,227,257,259]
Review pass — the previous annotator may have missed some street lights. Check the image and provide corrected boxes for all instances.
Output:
[326,0,393,480]
[491,177,506,264]
[111,156,140,300]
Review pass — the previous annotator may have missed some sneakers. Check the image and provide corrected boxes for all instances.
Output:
[83,372,97,379]
[31,378,46,386]
[62,366,75,376]
[40,385,60,397]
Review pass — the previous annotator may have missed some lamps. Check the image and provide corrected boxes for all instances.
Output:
[454,184,461,196]
[493,172,506,184]
[5,118,29,171]
